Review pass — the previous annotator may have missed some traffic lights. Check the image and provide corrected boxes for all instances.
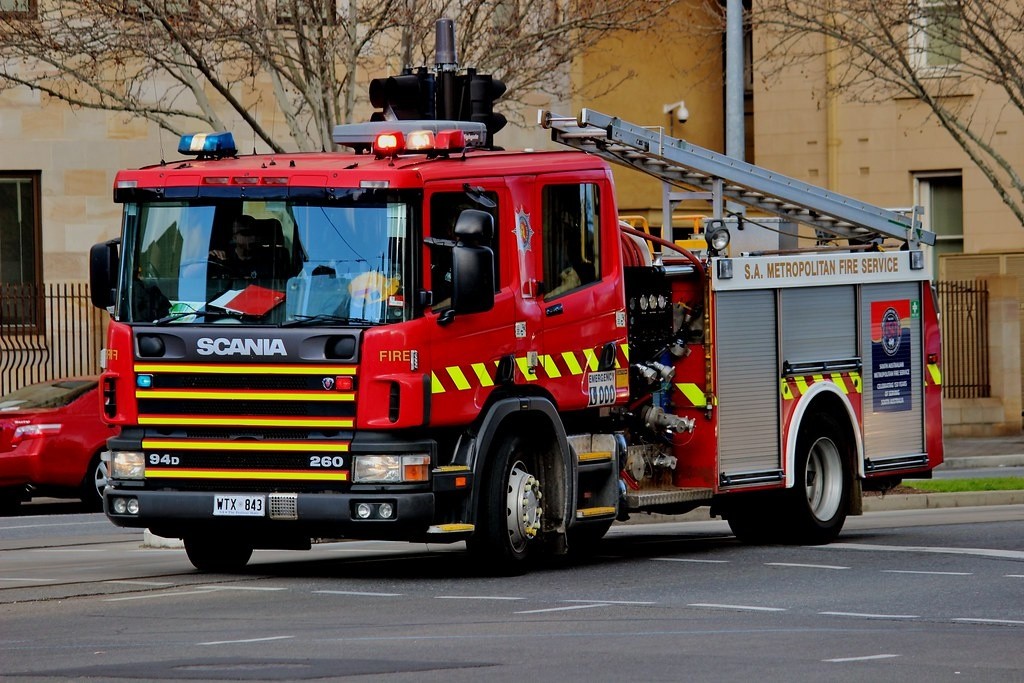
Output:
[369,65,434,122]
[454,67,508,152]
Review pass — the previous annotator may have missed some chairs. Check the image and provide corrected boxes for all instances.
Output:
[230,218,290,275]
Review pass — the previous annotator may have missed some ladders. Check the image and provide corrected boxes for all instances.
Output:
[538,108,938,246]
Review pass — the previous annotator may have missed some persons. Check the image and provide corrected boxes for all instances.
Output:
[209,216,301,318]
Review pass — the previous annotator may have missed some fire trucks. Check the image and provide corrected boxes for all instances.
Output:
[88,106,944,577]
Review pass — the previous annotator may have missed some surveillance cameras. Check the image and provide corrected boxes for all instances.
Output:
[677,108,690,123]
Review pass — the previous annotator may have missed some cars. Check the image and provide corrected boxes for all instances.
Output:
[0,374,121,515]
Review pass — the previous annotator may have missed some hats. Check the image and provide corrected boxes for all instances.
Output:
[231,216,256,235]
